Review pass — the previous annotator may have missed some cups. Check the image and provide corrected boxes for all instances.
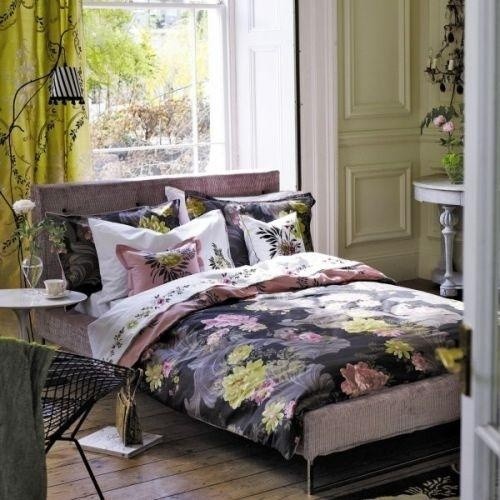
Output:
[42,278,68,294]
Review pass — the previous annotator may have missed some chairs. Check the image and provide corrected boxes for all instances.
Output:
[34,344,133,499]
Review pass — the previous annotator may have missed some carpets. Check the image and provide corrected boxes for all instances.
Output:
[309,447,460,499]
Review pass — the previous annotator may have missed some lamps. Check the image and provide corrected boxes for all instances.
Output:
[5,50,83,282]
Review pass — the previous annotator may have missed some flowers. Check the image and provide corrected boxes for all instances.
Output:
[421,104,464,172]
[11,198,60,270]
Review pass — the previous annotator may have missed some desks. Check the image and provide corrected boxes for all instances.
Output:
[0,287,87,346]
[412,171,465,299]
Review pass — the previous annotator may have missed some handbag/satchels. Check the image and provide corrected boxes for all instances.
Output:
[116,390,143,446]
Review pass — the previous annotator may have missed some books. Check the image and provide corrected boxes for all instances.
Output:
[73,425,164,459]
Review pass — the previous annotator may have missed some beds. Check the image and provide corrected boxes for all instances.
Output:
[27,169,465,494]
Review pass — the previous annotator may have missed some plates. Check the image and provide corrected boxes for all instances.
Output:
[41,290,71,298]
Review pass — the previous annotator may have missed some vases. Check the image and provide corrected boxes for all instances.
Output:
[444,161,461,183]
[22,256,43,287]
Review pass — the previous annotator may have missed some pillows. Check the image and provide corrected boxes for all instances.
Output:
[77,208,230,309]
[160,182,316,228]
[185,185,317,265]
[234,213,308,265]
[115,237,204,298]
[40,201,179,298]
